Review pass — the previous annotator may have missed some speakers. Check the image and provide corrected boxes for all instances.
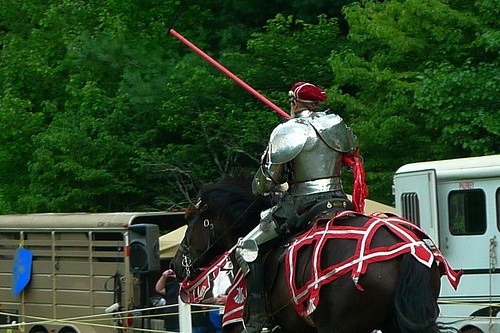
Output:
[127,223,160,275]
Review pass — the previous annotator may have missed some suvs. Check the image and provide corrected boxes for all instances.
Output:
[0,211,189,332]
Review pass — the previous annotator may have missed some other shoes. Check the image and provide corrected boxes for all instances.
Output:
[241,303,280,333]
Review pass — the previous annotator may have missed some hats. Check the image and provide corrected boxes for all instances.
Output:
[289,81,327,104]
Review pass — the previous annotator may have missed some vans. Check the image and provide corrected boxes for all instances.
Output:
[391,152,500,332]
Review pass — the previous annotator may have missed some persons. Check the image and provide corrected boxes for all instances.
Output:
[156,269,207,332]
[240,82,359,331]
[217,286,234,305]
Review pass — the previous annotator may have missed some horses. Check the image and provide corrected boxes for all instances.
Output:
[169,181,442,333]
